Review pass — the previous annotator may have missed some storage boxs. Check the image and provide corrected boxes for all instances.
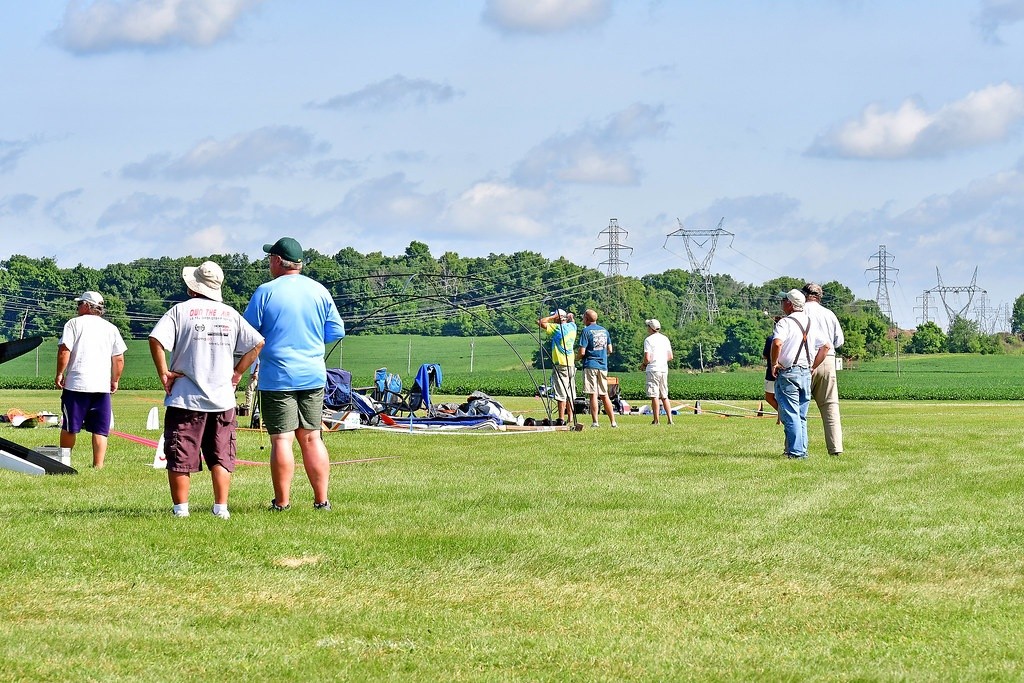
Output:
[38,448,71,467]
[332,411,360,430]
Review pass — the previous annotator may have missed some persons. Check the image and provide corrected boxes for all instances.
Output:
[763,316,781,425]
[784,283,844,457]
[640,319,673,425]
[56,292,128,468]
[538,309,577,425]
[577,310,617,427]
[771,290,830,460]
[149,260,265,520]
[241,236,345,510]
[246,356,260,408]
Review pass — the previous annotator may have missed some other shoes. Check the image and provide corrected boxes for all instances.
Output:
[591,423,599,427]
[212,510,230,520]
[314,500,331,511]
[268,499,291,512]
[170,510,189,517]
[612,424,618,427]
[831,453,838,456]
[652,421,655,424]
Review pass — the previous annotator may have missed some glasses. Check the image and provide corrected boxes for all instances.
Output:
[78,301,92,306]
[265,254,282,262]
[783,298,791,306]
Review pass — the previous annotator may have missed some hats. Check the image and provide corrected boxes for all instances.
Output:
[182,261,224,301]
[779,289,806,307]
[800,282,823,297]
[645,319,661,331]
[263,237,304,263]
[74,291,105,307]
[550,309,567,318]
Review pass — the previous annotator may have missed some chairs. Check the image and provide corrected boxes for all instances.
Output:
[323,363,443,426]
